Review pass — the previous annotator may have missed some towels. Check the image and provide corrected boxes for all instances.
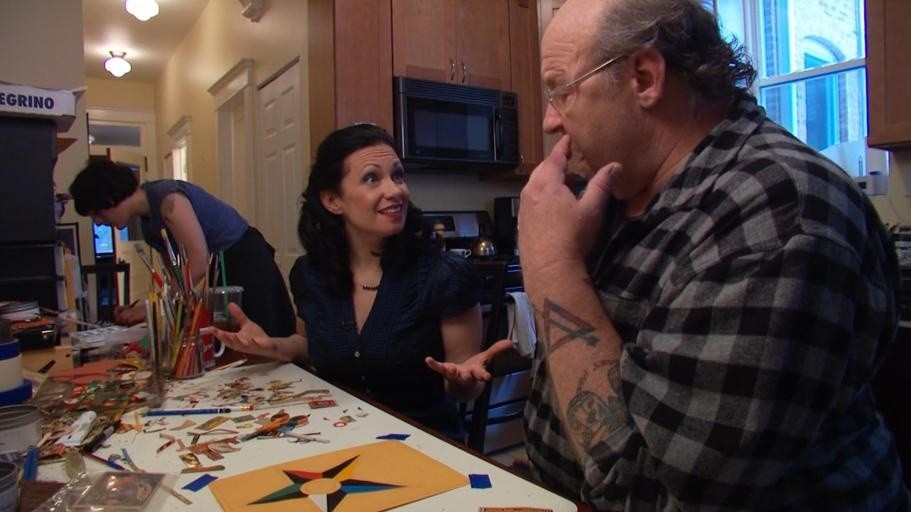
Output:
[505,291,537,361]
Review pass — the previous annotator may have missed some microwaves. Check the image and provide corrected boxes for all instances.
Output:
[391,75,521,177]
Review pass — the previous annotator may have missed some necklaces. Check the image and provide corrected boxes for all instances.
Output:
[362,283,381,291]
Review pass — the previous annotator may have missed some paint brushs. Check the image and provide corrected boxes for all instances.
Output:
[79,448,130,473]
[135,227,220,400]
[44,307,61,315]
[62,317,103,328]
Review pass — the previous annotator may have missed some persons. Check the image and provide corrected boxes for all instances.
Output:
[207,118,515,447]
[515,0,910,510]
[69,156,299,338]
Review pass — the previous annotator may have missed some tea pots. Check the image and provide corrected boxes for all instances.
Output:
[469,222,498,258]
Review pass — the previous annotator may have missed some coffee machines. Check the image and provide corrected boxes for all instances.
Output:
[496,196,521,260]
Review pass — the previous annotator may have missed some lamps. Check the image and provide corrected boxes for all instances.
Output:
[104,51,131,78]
[125,0,160,22]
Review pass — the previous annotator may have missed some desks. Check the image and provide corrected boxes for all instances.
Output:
[1,335,590,512]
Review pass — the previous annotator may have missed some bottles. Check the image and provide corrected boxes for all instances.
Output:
[434,223,448,252]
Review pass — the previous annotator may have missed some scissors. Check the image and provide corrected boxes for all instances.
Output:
[240,413,307,441]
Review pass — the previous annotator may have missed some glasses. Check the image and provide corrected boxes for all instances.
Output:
[544,52,623,117]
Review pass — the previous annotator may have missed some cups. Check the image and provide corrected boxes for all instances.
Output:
[145,286,243,380]
[1,340,24,393]
[0,404,42,512]
[450,248,472,260]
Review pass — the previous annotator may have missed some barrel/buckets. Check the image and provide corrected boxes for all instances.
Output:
[0,301,40,324]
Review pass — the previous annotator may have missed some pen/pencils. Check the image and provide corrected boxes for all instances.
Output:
[114,299,140,324]
[83,419,122,451]
[147,405,254,416]
[480,508,554,512]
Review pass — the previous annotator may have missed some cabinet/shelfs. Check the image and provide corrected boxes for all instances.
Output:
[309,1,394,168]
[390,0,510,93]
[479,0,544,183]
[865,0,911,151]
